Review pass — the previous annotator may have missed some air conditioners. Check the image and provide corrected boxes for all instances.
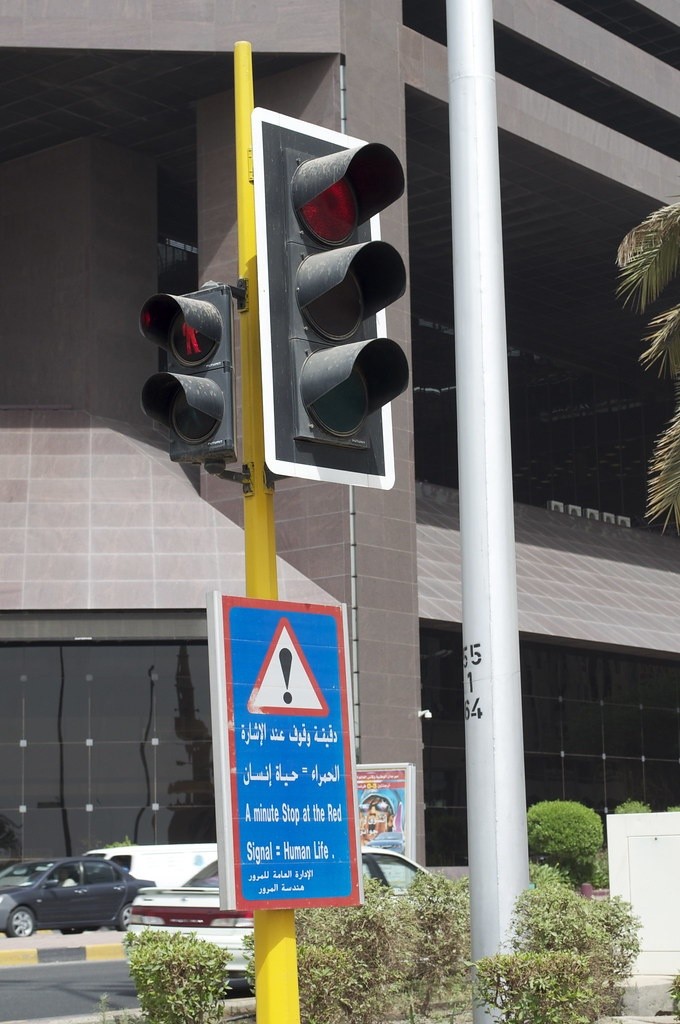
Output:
[599,512,615,524]
[615,516,630,527]
[547,500,564,512]
[564,504,581,517]
[583,508,599,520]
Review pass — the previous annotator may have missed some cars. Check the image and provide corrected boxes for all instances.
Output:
[130,845,438,994]
[0,856,155,937]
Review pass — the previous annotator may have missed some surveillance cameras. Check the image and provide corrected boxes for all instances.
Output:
[425,711,432,721]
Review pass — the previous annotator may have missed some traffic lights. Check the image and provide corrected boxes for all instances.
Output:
[139,284,237,464]
[251,107,413,494]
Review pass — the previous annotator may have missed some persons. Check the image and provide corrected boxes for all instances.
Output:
[51,864,79,887]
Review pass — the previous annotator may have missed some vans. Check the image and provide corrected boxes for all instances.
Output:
[84,843,218,891]
